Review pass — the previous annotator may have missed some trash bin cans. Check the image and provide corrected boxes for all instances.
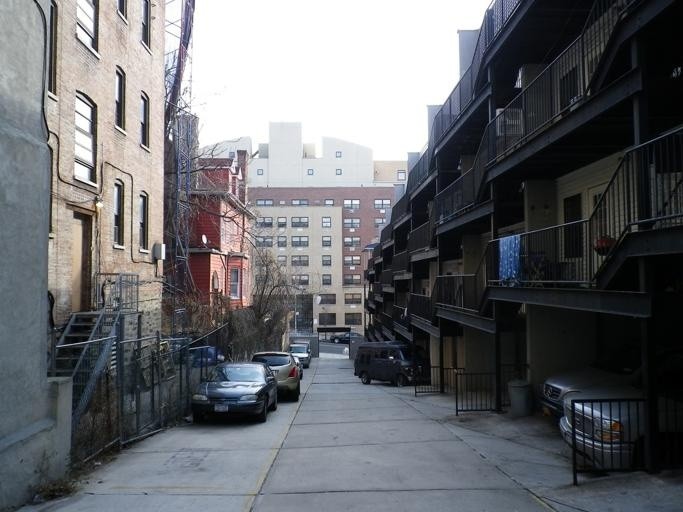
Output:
[508,379,530,417]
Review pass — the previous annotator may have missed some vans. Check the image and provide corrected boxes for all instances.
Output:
[353,341,431,387]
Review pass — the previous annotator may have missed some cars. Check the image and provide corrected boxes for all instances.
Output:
[191,361,278,424]
[540,360,646,475]
[249,339,312,402]
[330,332,364,344]
